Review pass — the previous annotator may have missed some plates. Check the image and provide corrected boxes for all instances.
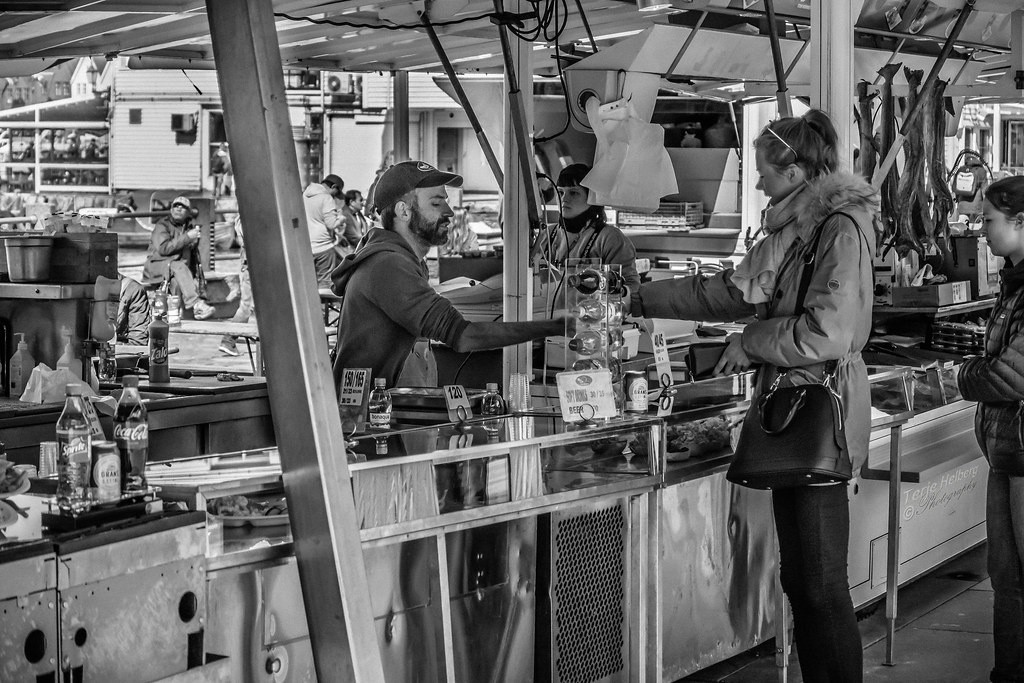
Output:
[204,502,290,527]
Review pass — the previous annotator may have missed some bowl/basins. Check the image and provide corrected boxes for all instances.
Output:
[4,236,52,282]
[593,439,690,459]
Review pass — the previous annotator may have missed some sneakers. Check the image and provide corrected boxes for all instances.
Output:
[193,300,216,320]
[219,339,243,356]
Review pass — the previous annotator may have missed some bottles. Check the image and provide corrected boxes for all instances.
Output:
[571,299,627,324]
[480,383,504,416]
[55,383,92,518]
[372,434,389,454]
[568,270,626,294]
[147,313,169,383]
[97,332,117,382]
[367,377,392,429]
[112,375,149,491]
[572,357,626,384]
[483,418,505,443]
[569,328,625,355]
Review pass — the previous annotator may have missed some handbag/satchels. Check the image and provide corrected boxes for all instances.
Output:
[726,383,853,490]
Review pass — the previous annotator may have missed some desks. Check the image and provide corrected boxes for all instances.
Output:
[318,287,344,351]
[167,320,340,378]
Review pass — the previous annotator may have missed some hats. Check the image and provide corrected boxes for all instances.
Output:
[373,161,463,215]
[325,174,345,200]
[172,196,190,210]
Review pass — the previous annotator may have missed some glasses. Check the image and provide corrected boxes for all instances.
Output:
[760,122,798,165]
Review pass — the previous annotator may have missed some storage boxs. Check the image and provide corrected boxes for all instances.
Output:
[891,280,972,307]
[0,231,118,284]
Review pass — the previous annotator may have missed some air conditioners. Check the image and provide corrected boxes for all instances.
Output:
[323,70,352,96]
[172,113,193,133]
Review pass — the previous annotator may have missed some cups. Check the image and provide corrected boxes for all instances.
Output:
[12,464,38,480]
[508,372,531,411]
[38,442,59,482]
[505,416,535,442]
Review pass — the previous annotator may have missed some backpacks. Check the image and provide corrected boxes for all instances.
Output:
[954,166,979,196]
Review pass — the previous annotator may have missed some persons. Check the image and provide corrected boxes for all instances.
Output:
[211,143,232,196]
[330,161,576,436]
[116,274,150,346]
[342,190,368,245]
[957,175,1024,683]
[302,174,346,285]
[620,108,878,683]
[142,196,216,320]
[532,163,639,294]
[218,214,254,357]
